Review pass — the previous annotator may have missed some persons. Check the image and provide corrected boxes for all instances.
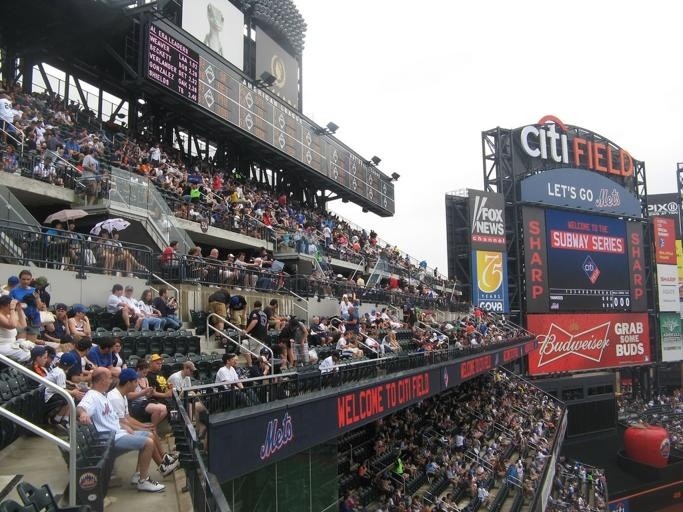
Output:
[72,337,94,383]
[287,315,311,366]
[544,457,607,511]
[112,339,126,371]
[65,224,96,270]
[47,220,64,266]
[342,368,560,511]
[252,354,273,398]
[318,352,340,388]
[0,78,113,185]
[1,295,35,363]
[263,300,284,332]
[208,248,223,281]
[69,306,90,342]
[87,337,112,387]
[235,253,257,291]
[35,277,50,306]
[158,241,185,281]
[47,303,70,342]
[76,366,180,491]
[1,276,18,294]
[83,148,99,205]
[155,286,180,331]
[267,332,292,371]
[31,345,48,377]
[208,290,232,333]
[213,355,254,406]
[186,248,206,277]
[12,272,35,303]
[167,361,210,420]
[106,285,140,331]
[96,230,115,272]
[225,253,238,281]
[311,292,448,356]
[253,257,266,290]
[137,288,164,331]
[448,305,527,349]
[145,355,166,402]
[105,368,182,474]
[123,286,144,330]
[229,295,247,328]
[115,122,265,238]
[128,360,166,438]
[46,354,83,429]
[246,300,265,343]
[265,186,459,310]
[108,232,151,275]
[617,383,681,450]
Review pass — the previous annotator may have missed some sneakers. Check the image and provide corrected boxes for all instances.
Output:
[163,451,180,463]
[48,417,60,425]
[131,472,140,483]
[137,477,166,491]
[156,459,179,476]
[57,420,69,433]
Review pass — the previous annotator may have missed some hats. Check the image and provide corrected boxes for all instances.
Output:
[150,354,163,362]
[57,303,67,310]
[8,276,20,284]
[112,284,123,290]
[73,304,88,313]
[36,276,50,286]
[60,352,76,367]
[222,354,235,360]
[184,361,197,371]
[120,368,139,381]
[227,254,235,257]
[60,334,79,344]
[261,347,270,353]
[125,285,133,291]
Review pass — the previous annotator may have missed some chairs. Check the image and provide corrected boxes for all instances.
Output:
[0,482,89,512]
[58,417,114,503]
[72,327,201,364]
[0,364,65,450]
[122,354,230,370]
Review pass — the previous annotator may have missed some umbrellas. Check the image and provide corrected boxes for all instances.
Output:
[89,217,130,234]
[42,208,87,222]
